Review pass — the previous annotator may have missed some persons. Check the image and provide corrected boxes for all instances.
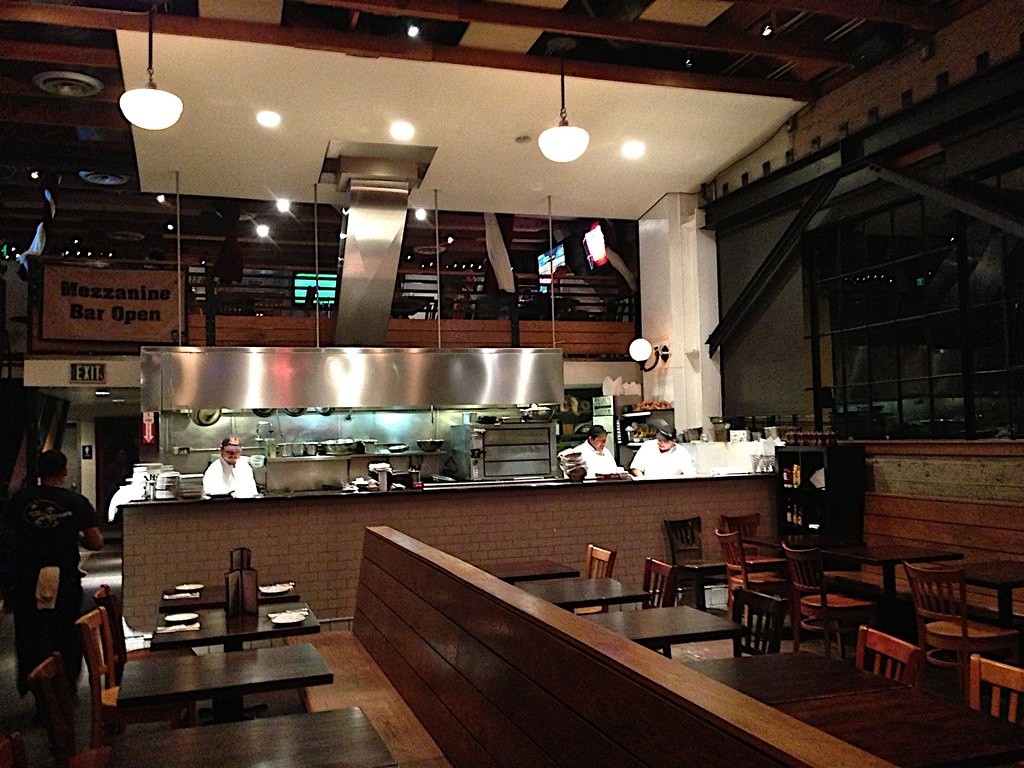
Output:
[628,425,697,477]
[550,266,603,319]
[0,450,105,716]
[564,425,617,479]
[203,435,258,495]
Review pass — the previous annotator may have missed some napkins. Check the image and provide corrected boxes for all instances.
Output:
[267,608,309,619]
[258,582,295,590]
[163,592,200,600]
[154,622,200,634]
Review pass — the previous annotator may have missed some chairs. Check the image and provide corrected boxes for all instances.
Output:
[0,585,200,768]
[574,513,1024,728]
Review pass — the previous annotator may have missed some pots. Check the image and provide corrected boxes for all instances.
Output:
[319,438,407,454]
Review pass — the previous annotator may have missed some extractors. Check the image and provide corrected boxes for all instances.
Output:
[140,344,564,412]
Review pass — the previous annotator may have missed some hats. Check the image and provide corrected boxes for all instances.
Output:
[37,449,68,476]
[656,425,677,442]
[220,436,243,452]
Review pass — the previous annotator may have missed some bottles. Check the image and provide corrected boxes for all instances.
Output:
[786,430,836,448]
[784,498,803,532]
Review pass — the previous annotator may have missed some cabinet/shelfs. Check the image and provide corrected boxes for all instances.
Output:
[775,444,866,548]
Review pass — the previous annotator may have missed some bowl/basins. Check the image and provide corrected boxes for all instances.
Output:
[519,408,553,423]
[277,441,318,456]
[432,474,456,483]
[416,437,445,453]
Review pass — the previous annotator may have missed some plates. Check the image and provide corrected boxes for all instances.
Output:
[271,615,305,624]
[175,584,204,590]
[164,613,199,623]
[260,585,290,594]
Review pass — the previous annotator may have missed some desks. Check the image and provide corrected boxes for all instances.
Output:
[579,605,749,649]
[105,706,398,767]
[150,602,320,651]
[472,560,651,609]
[116,643,334,728]
[924,559,1024,630]
[819,542,963,610]
[739,536,846,560]
[157,583,301,614]
[769,686,1024,768]
[679,651,912,706]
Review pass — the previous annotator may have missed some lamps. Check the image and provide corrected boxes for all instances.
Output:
[538,38,590,163]
[119,0,183,130]
[629,338,669,372]
